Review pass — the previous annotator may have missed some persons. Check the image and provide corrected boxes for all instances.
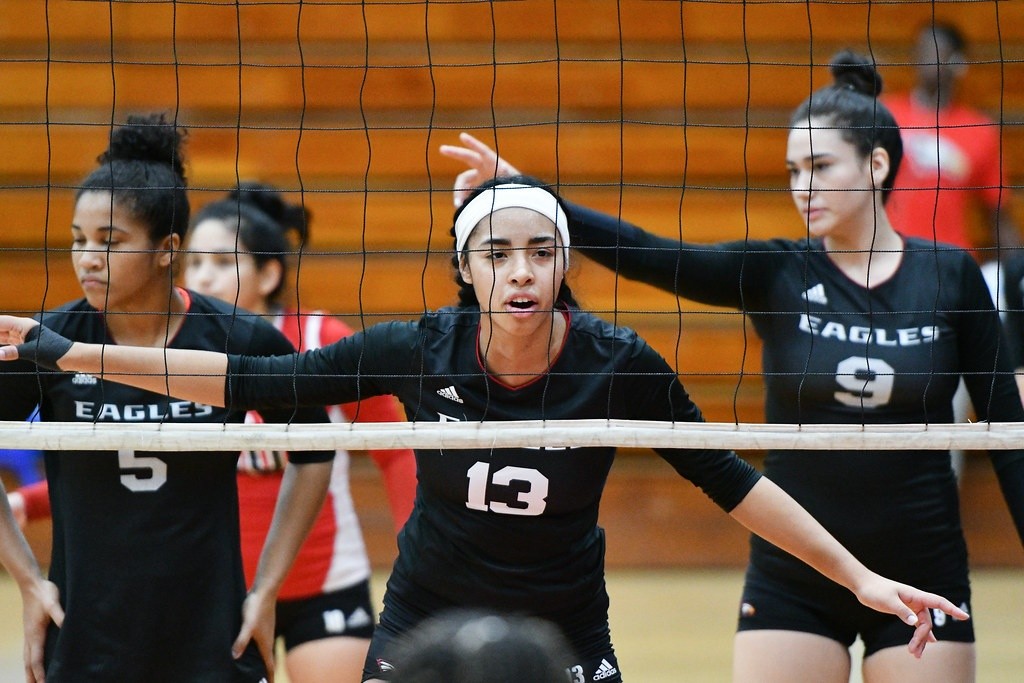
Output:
[438,17,1024,683]
[0,111,335,683]
[0,174,969,683]
[8,181,416,683]
